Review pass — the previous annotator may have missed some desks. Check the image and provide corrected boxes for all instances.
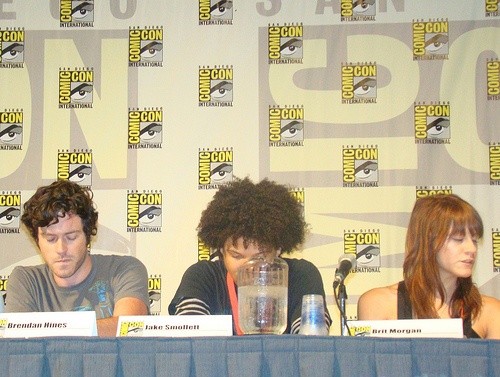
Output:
[0,335,500,377]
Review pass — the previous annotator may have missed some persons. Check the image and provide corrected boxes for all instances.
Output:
[5,181,148,337]
[358,194,500,339]
[168,177,332,336]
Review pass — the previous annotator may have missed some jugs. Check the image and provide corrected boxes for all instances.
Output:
[238,249,288,335]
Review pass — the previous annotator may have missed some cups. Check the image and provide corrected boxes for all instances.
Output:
[299,292,329,336]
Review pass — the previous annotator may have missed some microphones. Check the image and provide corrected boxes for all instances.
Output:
[333,253,355,289]
